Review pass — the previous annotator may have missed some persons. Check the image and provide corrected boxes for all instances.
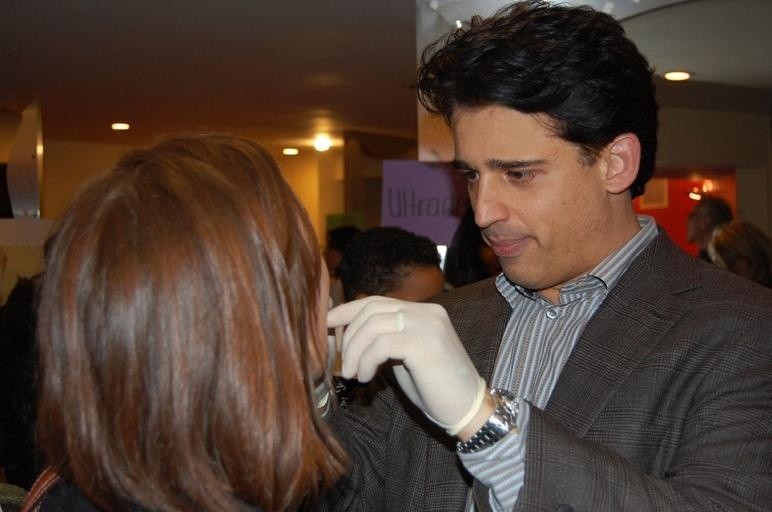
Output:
[324,210,502,303]
[688,196,771,287]
[313,0,771,512]
[24,132,373,506]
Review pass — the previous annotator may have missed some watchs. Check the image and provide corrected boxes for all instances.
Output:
[453,383,519,454]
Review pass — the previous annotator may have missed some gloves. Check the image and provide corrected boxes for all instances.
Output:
[323,292,489,442]
